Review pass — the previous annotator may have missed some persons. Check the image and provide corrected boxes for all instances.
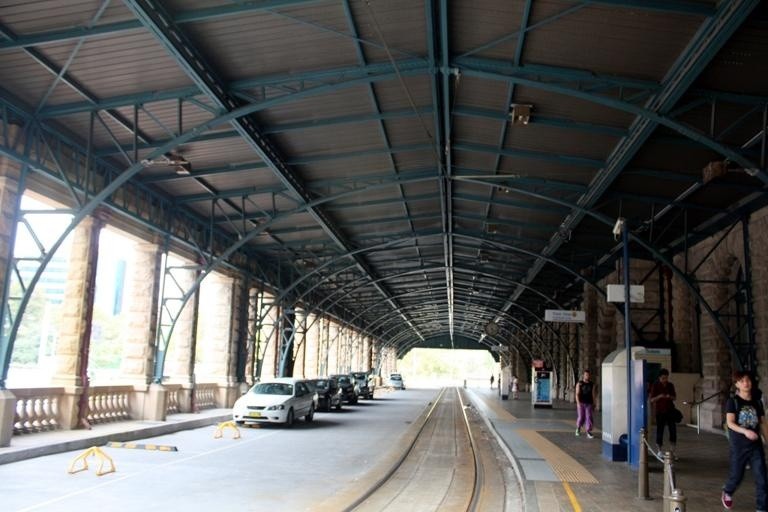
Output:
[721,370,768,512]
[574,370,596,438]
[511,375,519,400]
[649,368,679,461]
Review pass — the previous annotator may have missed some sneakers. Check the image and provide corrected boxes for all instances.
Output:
[576,428,580,436]
[672,452,679,460]
[587,433,594,438]
[721,491,733,509]
[658,452,663,458]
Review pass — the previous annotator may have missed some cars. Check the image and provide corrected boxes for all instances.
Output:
[307,372,375,410]
[388,372,403,388]
[232,372,318,428]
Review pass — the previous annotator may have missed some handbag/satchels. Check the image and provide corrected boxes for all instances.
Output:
[672,408,683,423]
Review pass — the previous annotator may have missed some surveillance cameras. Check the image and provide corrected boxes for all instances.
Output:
[612,217,625,234]
